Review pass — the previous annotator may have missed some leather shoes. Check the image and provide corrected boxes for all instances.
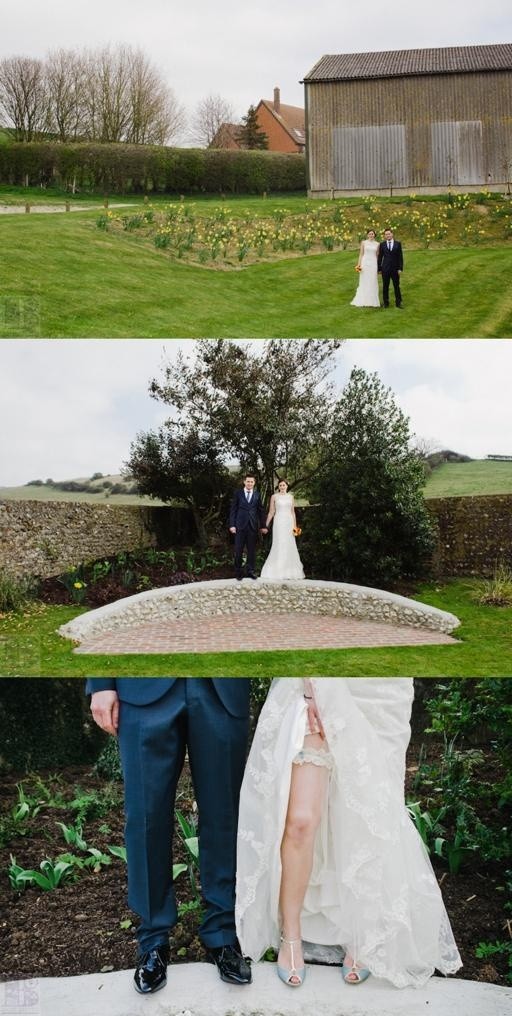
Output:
[205,946,252,983]
[384,303,404,309]
[236,570,257,580]
[134,944,170,994]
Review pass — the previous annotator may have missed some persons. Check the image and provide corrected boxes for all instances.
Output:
[85,676,415,995]
[228,473,305,581]
[350,228,405,309]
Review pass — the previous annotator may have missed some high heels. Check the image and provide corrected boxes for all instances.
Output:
[276,937,306,987]
[342,948,370,984]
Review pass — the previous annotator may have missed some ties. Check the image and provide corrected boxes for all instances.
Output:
[247,490,251,502]
[389,242,391,252]
